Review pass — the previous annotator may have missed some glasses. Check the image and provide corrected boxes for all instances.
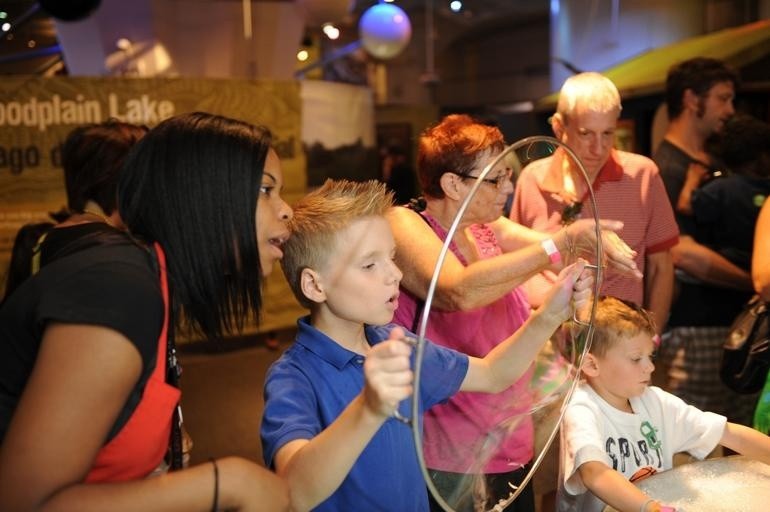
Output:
[457,166,514,190]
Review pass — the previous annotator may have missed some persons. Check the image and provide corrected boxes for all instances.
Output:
[384,114,644,512]
[557,296,768,511]
[651,57,769,469]
[509,72,681,511]
[260,178,593,511]
[4,111,294,511]
[379,143,421,206]
[4,118,151,300]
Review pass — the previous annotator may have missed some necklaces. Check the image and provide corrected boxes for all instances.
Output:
[84,209,114,226]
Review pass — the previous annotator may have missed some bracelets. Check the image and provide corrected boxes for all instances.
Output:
[208,457,219,510]
[640,498,661,510]
[542,238,562,264]
[661,506,676,511]
[563,224,576,252]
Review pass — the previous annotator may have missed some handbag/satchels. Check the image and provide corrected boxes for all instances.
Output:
[721,294,770,394]
